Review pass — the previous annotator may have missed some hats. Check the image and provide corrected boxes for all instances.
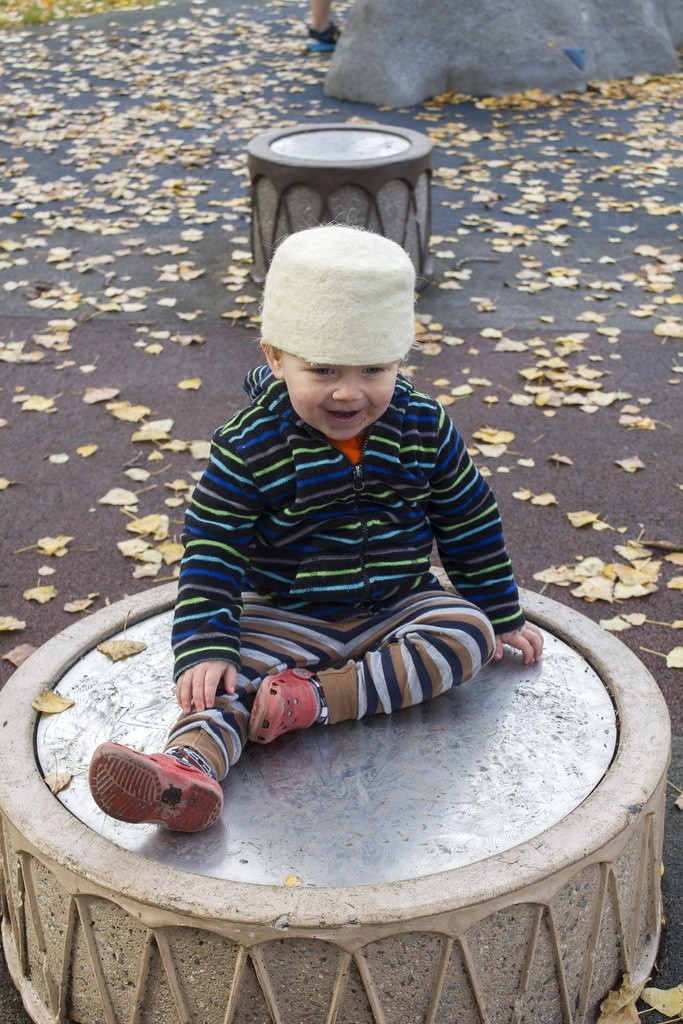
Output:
[261,225,415,366]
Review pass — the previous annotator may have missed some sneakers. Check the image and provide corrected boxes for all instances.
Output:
[302,22,339,52]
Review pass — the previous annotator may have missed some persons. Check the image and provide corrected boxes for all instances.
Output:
[306,0,341,53]
[91,221,546,831]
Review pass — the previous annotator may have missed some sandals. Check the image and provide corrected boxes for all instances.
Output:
[90,742,223,832]
[248,668,316,744]
